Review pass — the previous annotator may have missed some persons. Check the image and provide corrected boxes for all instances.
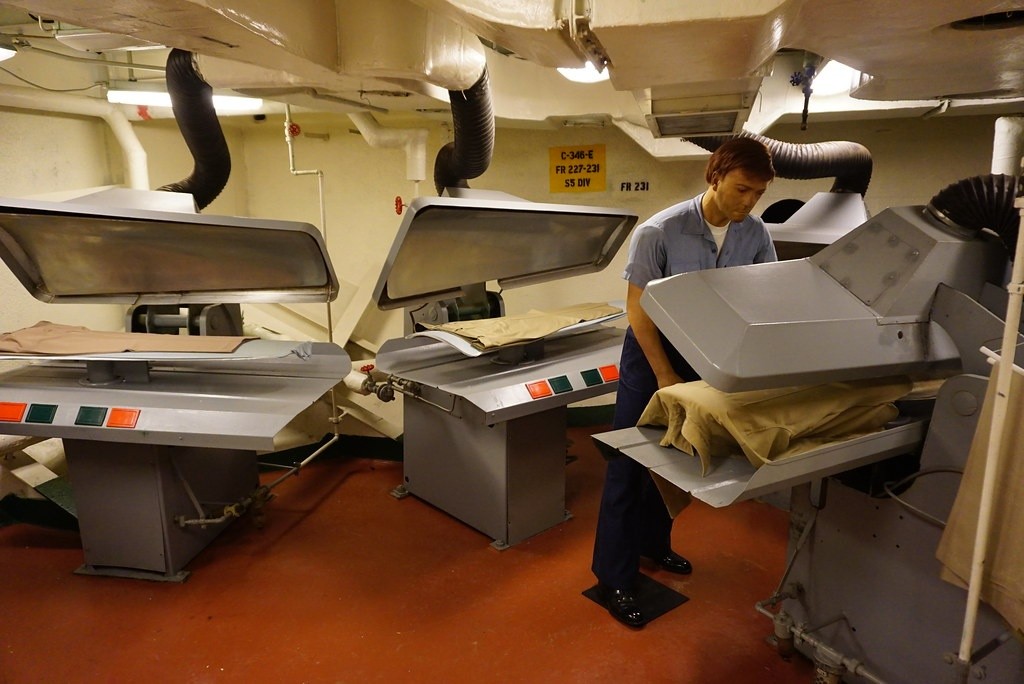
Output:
[591,138,777,620]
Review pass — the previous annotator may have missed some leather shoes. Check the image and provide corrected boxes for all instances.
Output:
[598,581,645,626]
[641,548,691,574]
